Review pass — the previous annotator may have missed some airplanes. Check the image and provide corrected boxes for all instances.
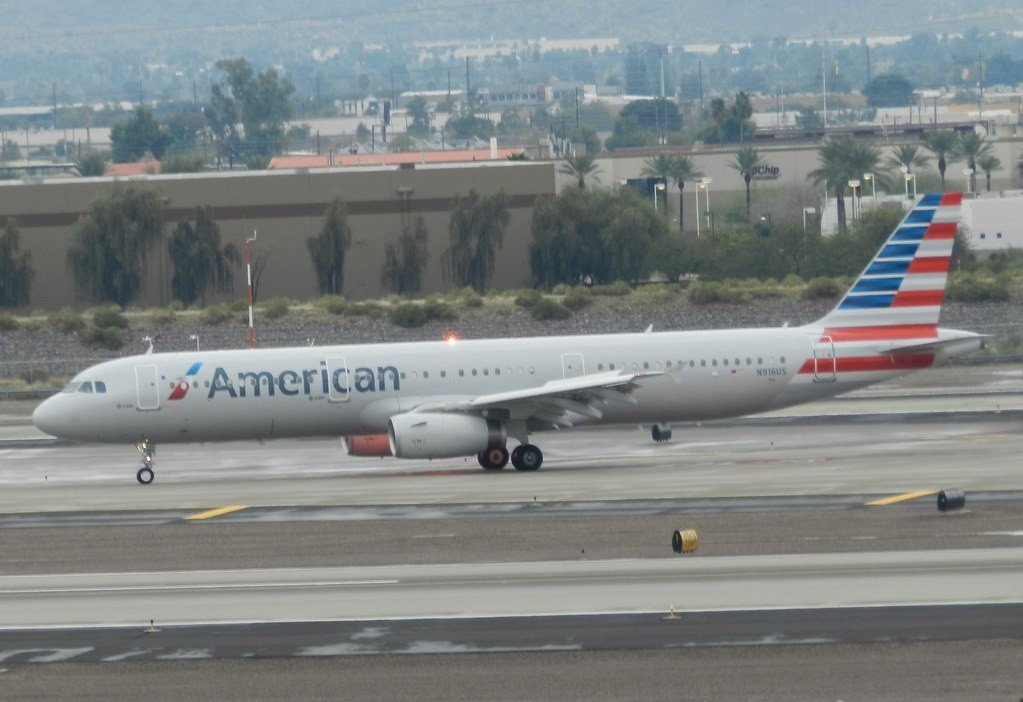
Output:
[29,191,997,486]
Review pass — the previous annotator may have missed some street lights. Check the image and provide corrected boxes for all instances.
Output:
[653,184,666,212]
[904,174,917,200]
[900,165,909,196]
[863,173,876,205]
[963,168,972,191]
[695,182,705,237]
[701,176,713,233]
[849,179,861,223]
